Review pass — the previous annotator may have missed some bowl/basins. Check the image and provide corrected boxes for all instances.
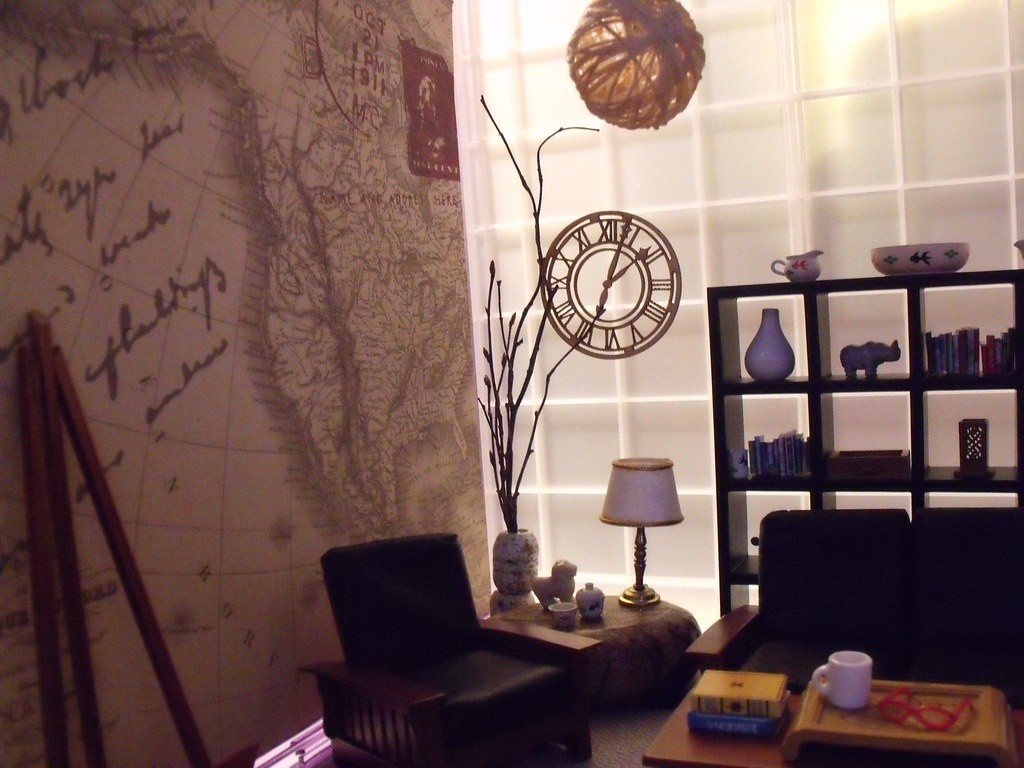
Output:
[868,241,970,274]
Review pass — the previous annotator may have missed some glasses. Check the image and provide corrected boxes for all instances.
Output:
[877,686,971,731]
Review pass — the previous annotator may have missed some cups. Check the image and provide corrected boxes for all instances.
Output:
[547,603,578,627]
[575,582,605,620]
[728,448,749,480]
[811,650,874,710]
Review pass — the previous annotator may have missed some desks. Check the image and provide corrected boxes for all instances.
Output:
[702,268,1023,617]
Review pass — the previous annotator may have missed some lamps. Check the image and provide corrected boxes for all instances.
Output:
[599,457,688,609]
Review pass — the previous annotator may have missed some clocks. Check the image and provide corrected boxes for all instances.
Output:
[540,208,682,361]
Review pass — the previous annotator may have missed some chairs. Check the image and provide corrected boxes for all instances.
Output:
[293,532,603,768]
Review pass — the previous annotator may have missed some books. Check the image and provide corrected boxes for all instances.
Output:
[692,670,789,717]
[687,706,793,738]
[748,430,809,476]
[925,327,1015,375]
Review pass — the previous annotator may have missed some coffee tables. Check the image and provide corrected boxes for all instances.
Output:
[640,671,1024,768]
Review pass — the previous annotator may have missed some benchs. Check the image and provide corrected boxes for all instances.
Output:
[685,508,1024,708]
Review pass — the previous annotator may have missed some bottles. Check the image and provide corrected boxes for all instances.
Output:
[744,307,796,381]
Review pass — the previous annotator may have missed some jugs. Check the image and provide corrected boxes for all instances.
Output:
[768,249,825,282]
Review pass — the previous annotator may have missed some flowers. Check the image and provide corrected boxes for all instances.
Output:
[473,93,606,532]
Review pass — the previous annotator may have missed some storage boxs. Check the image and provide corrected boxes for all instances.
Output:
[825,451,910,481]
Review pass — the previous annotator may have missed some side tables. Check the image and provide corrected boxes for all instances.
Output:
[487,594,704,706]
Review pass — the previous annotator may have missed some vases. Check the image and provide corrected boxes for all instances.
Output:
[743,308,799,380]
[494,529,541,595]
